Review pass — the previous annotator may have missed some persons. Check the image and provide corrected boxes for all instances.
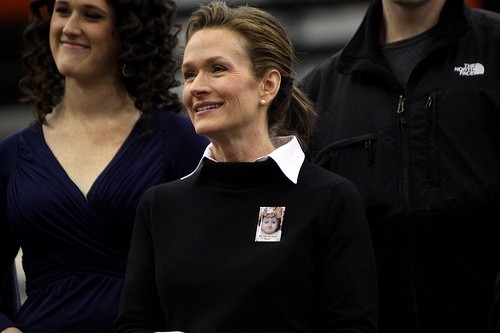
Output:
[277,0,500,332]
[114,0,380,332]
[258,206,283,242]
[1,0,210,333]
